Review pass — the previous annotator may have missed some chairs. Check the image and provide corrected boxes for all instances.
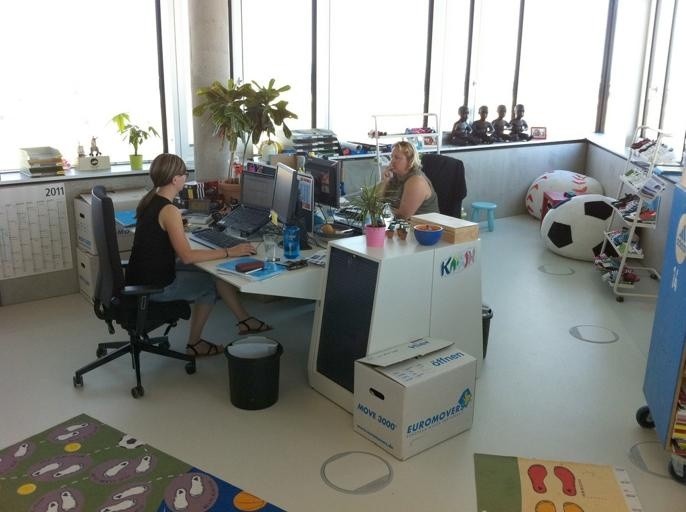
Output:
[418,150,469,220]
[72,183,197,402]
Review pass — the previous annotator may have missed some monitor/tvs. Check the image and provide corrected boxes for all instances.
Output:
[304,158,339,208]
[274,162,300,234]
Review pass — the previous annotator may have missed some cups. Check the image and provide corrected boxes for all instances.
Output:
[283,227,300,260]
[261,233,279,263]
[424,137,433,145]
[407,137,419,149]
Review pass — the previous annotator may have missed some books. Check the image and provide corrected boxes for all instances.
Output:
[179,180,205,200]
[114,209,137,227]
[215,257,287,281]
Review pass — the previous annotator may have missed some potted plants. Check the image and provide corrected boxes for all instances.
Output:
[108,111,160,172]
[190,75,298,208]
[341,168,401,248]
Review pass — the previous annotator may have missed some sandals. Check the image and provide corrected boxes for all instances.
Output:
[237,316,274,335]
[186,339,226,357]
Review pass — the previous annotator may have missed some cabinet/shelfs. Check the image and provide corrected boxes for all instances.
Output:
[633,180,686,486]
[371,109,443,185]
[594,122,686,306]
[304,228,485,417]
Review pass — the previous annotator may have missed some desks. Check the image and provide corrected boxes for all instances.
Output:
[126,207,328,301]
[318,195,373,231]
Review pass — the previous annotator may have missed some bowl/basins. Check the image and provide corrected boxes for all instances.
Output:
[414,224,445,246]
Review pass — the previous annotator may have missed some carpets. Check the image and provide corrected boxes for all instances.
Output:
[1,410,291,511]
[469,450,646,512]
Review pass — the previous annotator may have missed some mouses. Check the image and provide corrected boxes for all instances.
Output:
[242,250,258,257]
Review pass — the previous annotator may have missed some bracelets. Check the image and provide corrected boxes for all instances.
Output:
[224,247,228,258]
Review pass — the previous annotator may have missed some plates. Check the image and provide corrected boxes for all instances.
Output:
[312,223,353,238]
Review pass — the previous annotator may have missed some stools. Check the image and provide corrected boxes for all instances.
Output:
[469,201,497,234]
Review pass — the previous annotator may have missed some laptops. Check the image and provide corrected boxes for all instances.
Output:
[216,171,275,235]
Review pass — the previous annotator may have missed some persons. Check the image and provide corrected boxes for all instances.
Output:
[471,106,495,144]
[124,153,275,358]
[509,104,534,141]
[375,142,441,220]
[448,106,473,146]
[492,105,511,142]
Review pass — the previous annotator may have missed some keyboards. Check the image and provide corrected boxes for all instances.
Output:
[189,228,250,250]
[332,209,369,229]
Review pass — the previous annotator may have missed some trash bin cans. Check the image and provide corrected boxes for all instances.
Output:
[482,305,493,360]
[224,336,284,410]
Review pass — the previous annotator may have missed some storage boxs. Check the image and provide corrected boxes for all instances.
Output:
[539,190,587,226]
[350,335,478,463]
[408,211,480,245]
[72,243,133,307]
[72,186,150,257]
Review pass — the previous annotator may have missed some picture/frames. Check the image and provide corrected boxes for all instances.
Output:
[530,126,547,141]
